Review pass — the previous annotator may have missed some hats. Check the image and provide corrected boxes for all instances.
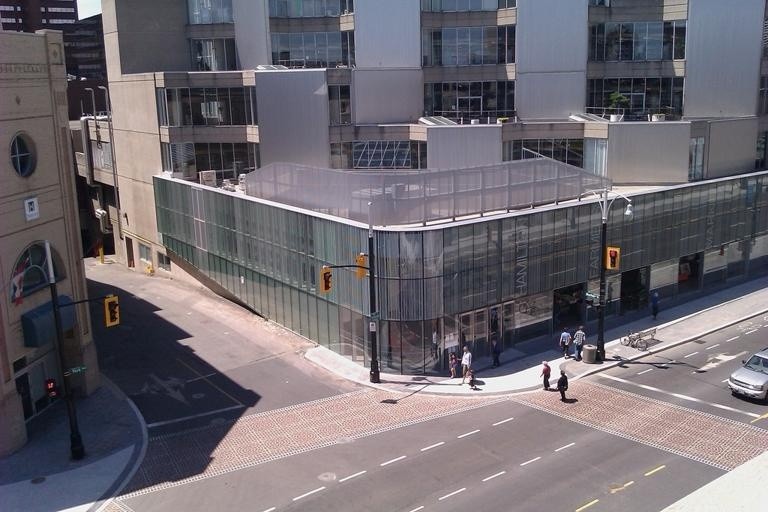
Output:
[561,370,565,374]
[542,361,548,364]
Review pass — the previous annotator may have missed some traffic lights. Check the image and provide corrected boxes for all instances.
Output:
[103,295,120,327]
[606,247,619,271]
[44,377,60,400]
[319,266,333,294]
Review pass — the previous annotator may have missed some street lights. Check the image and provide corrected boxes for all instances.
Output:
[579,184,634,341]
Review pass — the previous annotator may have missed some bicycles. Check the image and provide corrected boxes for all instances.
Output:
[620,328,647,352]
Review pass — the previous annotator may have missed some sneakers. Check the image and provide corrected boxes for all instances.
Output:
[451,375,456,378]
[543,388,548,390]
[561,398,566,402]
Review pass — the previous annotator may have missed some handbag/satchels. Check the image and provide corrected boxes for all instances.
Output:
[468,374,476,381]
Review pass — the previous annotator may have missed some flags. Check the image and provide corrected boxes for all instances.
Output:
[13,257,30,305]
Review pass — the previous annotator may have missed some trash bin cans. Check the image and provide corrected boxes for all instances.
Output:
[582,344,596,364]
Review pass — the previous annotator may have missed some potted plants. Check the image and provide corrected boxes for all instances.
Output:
[608,90,630,122]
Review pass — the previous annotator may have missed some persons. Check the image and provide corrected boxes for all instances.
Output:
[573,325,586,361]
[459,345,473,385]
[450,353,459,379]
[433,328,440,356]
[559,326,573,359]
[490,339,501,370]
[648,292,661,321]
[468,369,476,390]
[556,370,569,402]
[540,360,551,390]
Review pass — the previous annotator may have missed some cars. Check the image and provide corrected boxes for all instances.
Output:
[727,345,768,400]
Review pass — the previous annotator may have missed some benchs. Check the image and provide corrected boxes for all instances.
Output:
[635,327,656,343]
